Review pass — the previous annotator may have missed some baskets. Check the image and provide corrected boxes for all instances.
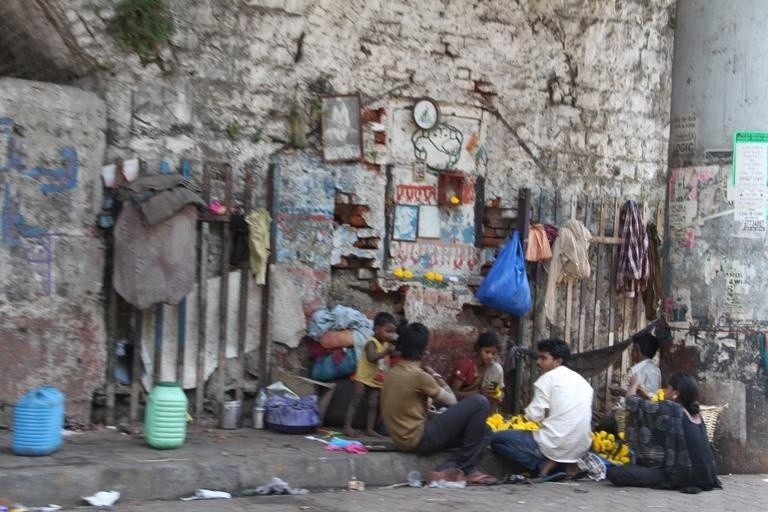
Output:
[698,404,726,444]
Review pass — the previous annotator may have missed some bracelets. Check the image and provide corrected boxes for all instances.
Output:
[430,371,445,379]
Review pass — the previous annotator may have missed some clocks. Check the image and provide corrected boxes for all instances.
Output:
[413,96,439,130]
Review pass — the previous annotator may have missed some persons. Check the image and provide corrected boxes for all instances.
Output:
[487,336,596,483]
[606,366,725,494]
[453,327,506,415]
[593,332,663,441]
[377,321,499,488]
[342,310,397,439]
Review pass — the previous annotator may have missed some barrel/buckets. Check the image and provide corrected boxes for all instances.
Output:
[145,380,189,447]
[13,387,66,453]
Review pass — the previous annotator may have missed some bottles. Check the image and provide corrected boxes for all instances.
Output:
[252,387,268,429]
[218,399,243,430]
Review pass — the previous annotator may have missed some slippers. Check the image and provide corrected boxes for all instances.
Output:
[530,468,592,483]
[423,466,495,486]
